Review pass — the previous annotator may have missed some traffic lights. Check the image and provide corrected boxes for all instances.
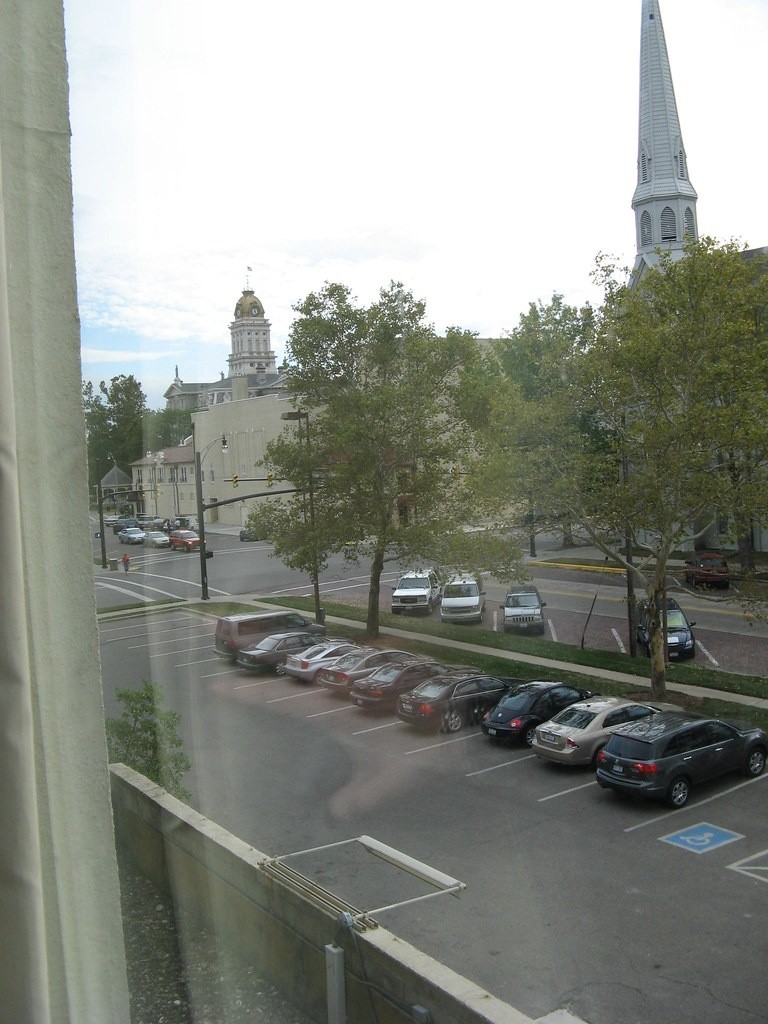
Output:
[231,473,238,488]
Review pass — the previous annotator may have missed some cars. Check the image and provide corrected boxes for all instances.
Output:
[316,646,422,693]
[240,527,268,541]
[235,631,356,677]
[150,518,173,532]
[116,527,146,545]
[532,697,686,772]
[396,669,527,733]
[143,531,170,548]
[285,640,362,689]
[103,511,162,536]
[481,679,602,749]
[348,657,484,714]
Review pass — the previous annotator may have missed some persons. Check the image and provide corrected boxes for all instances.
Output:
[122,553,129,573]
[175,518,180,529]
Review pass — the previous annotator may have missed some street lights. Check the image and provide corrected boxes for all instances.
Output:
[106,451,119,514]
[280,405,324,626]
[195,432,230,600]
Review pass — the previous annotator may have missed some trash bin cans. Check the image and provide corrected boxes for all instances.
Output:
[109,558,118,571]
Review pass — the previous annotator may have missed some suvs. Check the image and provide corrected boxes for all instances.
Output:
[595,710,768,809]
[391,567,444,615]
[436,569,487,624]
[213,608,326,662]
[634,597,696,659]
[500,585,547,634]
[170,530,206,554]
[684,551,732,589]
